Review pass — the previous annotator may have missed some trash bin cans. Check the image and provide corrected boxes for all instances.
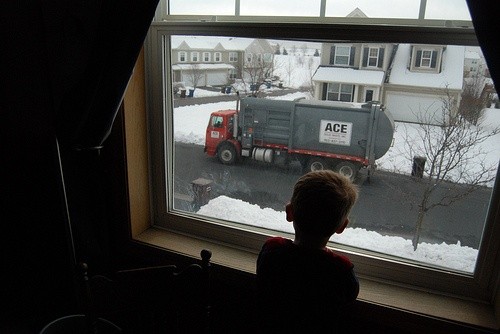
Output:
[411,155,428,179]
[267,83,271,88]
[279,84,282,88]
[188,89,194,97]
[221,86,226,93]
[254,85,257,91]
[250,85,254,91]
[226,86,231,94]
[180,90,186,98]
[190,178,212,211]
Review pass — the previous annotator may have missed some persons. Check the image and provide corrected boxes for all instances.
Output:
[255,170,360,333]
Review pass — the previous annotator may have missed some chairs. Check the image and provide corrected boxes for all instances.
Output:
[75,250,214,334]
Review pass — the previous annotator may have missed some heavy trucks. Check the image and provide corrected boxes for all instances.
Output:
[202,91,395,183]
